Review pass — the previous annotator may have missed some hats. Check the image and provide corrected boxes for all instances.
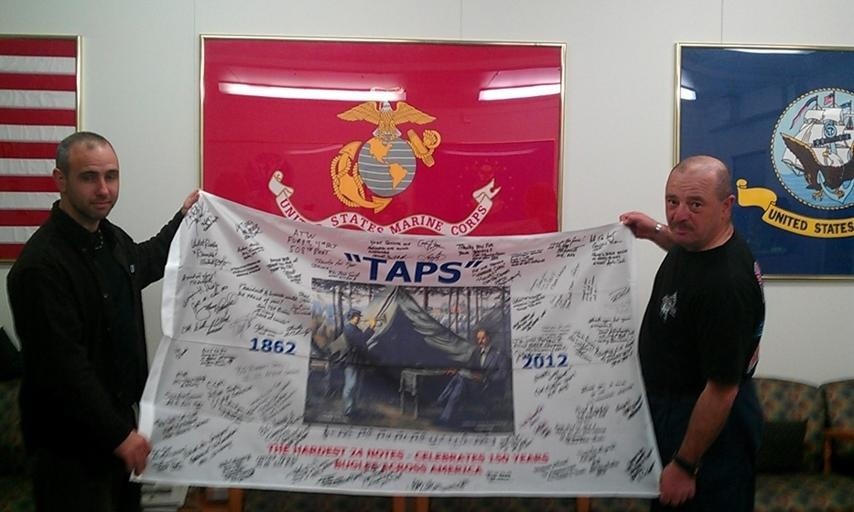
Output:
[347,309,363,320]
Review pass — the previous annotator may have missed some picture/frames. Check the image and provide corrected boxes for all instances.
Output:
[675,42,854,279]
[1,33,83,265]
[200,35,568,236]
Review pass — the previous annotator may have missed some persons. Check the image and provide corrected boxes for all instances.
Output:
[616,154,766,511]
[431,326,507,424]
[341,308,377,418]
[1,130,204,510]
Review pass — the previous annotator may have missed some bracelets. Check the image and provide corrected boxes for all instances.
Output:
[653,222,662,234]
[672,452,700,477]
